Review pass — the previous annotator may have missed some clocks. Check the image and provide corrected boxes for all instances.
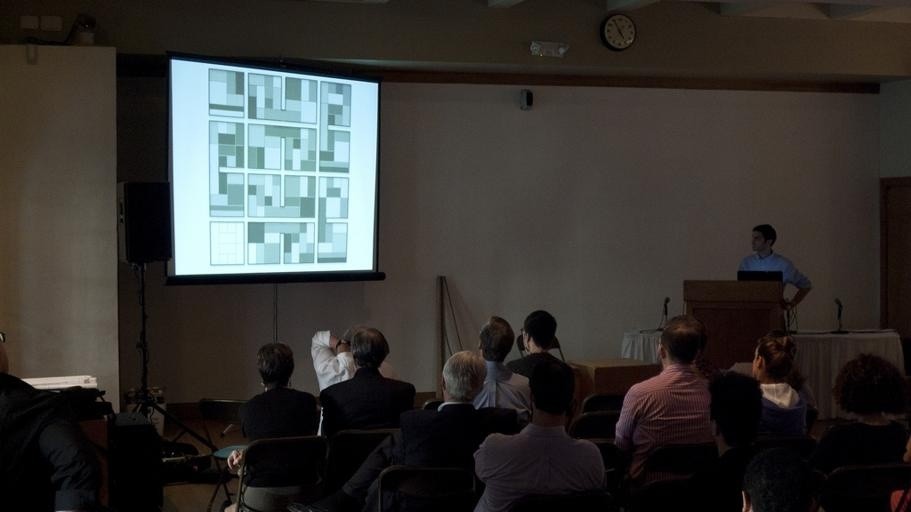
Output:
[601,14,638,51]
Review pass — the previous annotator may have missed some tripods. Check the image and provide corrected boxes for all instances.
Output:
[119,285,220,454]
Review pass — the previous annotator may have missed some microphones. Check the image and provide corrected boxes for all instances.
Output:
[657,296,671,331]
[830,298,849,334]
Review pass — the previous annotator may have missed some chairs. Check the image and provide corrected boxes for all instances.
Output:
[568,410,623,435]
[766,401,810,432]
[333,428,403,471]
[377,462,478,511]
[583,437,635,467]
[235,434,328,511]
[578,393,628,409]
[517,331,566,363]
[499,486,619,511]
[199,398,254,510]
[425,399,442,411]
[464,407,519,442]
[641,439,718,470]
[823,462,911,512]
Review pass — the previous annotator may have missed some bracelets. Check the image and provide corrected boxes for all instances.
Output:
[787,301,796,309]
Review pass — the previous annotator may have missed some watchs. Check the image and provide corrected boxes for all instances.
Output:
[335,339,351,349]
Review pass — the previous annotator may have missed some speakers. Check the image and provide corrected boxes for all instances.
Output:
[106,412,163,510]
[117,181,173,267]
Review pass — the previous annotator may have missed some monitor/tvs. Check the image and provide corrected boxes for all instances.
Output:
[737,270,783,281]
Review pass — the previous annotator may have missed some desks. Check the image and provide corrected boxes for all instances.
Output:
[571,355,663,393]
[618,316,903,420]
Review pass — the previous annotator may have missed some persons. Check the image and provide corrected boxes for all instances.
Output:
[472,316,533,422]
[320,326,417,455]
[503,310,575,383]
[691,368,771,512]
[286,349,532,512]
[0,331,107,512]
[311,329,394,436]
[227,342,319,511]
[613,312,718,487]
[736,223,815,312]
[740,332,911,512]
[472,361,609,512]
